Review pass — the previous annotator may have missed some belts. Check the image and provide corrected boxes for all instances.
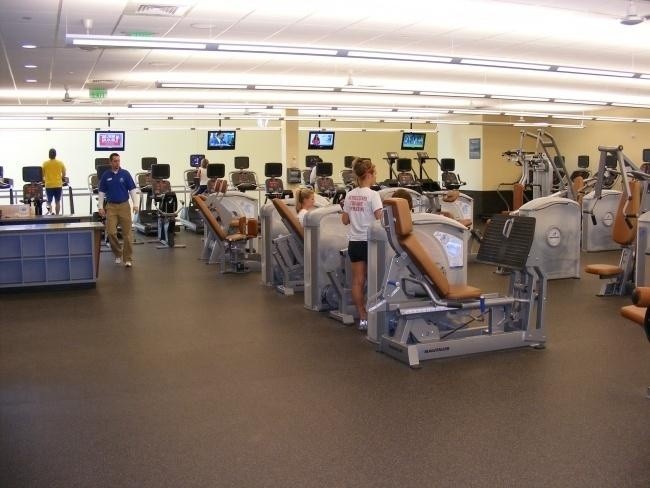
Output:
[112,202,121,204]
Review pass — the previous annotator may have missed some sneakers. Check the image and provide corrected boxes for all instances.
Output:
[124,262,133,267]
[358,321,369,332]
[116,258,120,263]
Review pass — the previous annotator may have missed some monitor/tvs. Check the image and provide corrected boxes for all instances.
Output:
[95,131,125,151]
[308,132,335,150]
[207,131,236,150]
[401,133,426,150]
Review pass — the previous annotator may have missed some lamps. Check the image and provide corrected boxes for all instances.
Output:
[0,1,650,127]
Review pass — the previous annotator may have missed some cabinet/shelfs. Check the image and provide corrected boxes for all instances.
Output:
[0,220,106,291]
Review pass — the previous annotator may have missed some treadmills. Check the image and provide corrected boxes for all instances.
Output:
[87,154,206,234]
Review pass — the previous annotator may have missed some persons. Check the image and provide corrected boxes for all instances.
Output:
[98,153,140,268]
[294,188,315,229]
[41,148,66,217]
[309,157,323,192]
[192,158,212,196]
[427,189,473,229]
[312,134,320,145]
[210,132,226,145]
[389,188,414,211]
[341,157,384,323]
